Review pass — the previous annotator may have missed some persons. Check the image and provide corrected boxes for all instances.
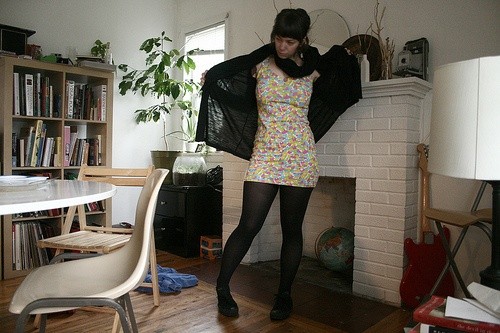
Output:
[194,8,362,320]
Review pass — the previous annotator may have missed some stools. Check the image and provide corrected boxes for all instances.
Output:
[200,234,223,261]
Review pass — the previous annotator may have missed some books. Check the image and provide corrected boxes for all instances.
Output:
[13,72,62,117]
[64,124,103,166]
[12,119,61,167]
[84,201,100,212]
[16,172,58,218]
[413,296,500,333]
[66,79,107,122]
[72,220,106,232]
[12,221,61,271]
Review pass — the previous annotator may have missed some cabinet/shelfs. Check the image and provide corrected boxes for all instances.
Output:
[154,186,223,257]
[0,55,114,281]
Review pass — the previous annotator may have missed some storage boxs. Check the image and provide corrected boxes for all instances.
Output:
[1,23,37,55]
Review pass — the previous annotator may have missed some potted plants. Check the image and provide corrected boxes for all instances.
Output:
[162,79,206,153]
[118,32,205,185]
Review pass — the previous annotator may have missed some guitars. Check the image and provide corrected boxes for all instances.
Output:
[399,144,457,310]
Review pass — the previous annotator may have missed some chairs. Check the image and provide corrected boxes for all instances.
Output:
[424,182,494,299]
[9,168,170,332]
[36,163,160,332]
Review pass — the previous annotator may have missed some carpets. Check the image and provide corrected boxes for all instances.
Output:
[177,259,402,333]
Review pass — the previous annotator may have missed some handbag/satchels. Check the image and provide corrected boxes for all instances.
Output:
[206,164,223,192]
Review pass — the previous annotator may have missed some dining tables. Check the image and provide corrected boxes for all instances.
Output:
[0,178,116,214]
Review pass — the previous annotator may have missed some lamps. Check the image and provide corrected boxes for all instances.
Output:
[426,56,500,290]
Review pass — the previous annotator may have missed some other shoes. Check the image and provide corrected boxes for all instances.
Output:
[215,286,238,317]
[270,292,294,321]
